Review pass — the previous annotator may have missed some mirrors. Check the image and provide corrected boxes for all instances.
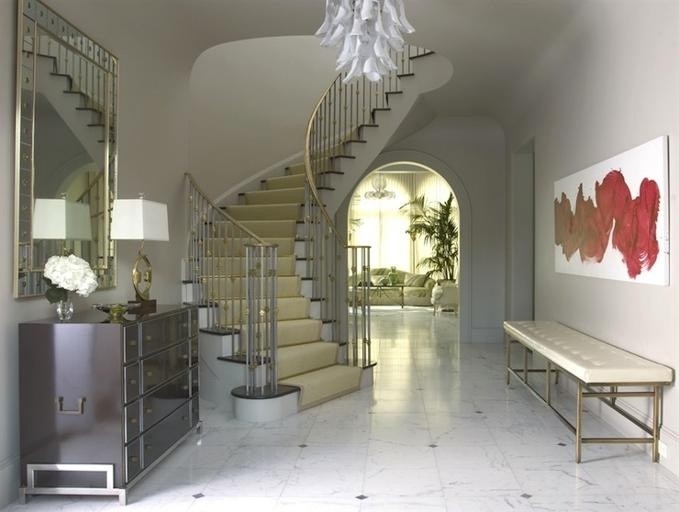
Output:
[13,0,119,300]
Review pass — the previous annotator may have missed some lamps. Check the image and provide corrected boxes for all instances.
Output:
[32,193,93,257]
[313,0,415,83]
[109,192,170,314]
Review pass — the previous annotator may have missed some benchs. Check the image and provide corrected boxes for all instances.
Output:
[503,320,675,464]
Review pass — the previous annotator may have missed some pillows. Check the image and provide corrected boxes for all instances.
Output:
[404,273,425,287]
[370,276,385,287]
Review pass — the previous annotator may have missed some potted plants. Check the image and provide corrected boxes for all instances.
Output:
[404,191,459,285]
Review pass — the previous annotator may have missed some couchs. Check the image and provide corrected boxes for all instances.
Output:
[346,268,436,306]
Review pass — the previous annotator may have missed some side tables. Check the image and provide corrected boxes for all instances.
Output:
[431,285,459,317]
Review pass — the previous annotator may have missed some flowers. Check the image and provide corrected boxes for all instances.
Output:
[42,254,99,304]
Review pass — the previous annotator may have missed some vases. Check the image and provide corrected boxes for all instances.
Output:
[56,292,74,320]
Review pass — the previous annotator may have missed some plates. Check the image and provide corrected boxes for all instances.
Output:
[90,302,143,318]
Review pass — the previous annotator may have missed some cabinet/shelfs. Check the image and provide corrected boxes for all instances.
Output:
[18,304,204,506]
